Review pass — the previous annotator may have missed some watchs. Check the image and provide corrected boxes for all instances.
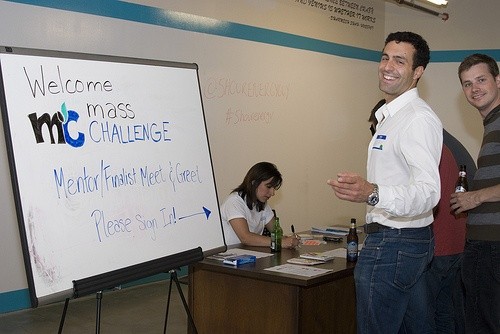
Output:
[366,184,380,205]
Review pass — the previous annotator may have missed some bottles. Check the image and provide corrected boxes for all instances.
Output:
[454,164,468,193]
[346,218,358,262]
[270,217,283,253]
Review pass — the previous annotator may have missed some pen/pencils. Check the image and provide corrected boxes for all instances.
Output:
[291,224,297,239]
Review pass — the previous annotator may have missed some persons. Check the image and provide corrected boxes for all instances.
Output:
[219,162,302,249]
[448,53,499,334]
[326,31,477,334]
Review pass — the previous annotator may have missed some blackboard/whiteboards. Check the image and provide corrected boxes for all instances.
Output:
[0,46,228,307]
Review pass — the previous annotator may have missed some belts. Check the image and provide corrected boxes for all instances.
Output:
[364,222,390,233]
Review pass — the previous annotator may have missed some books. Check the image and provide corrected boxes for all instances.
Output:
[300,252,337,262]
[312,225,350,236]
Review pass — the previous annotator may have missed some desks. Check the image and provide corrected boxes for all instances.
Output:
[187,224,366,334]
